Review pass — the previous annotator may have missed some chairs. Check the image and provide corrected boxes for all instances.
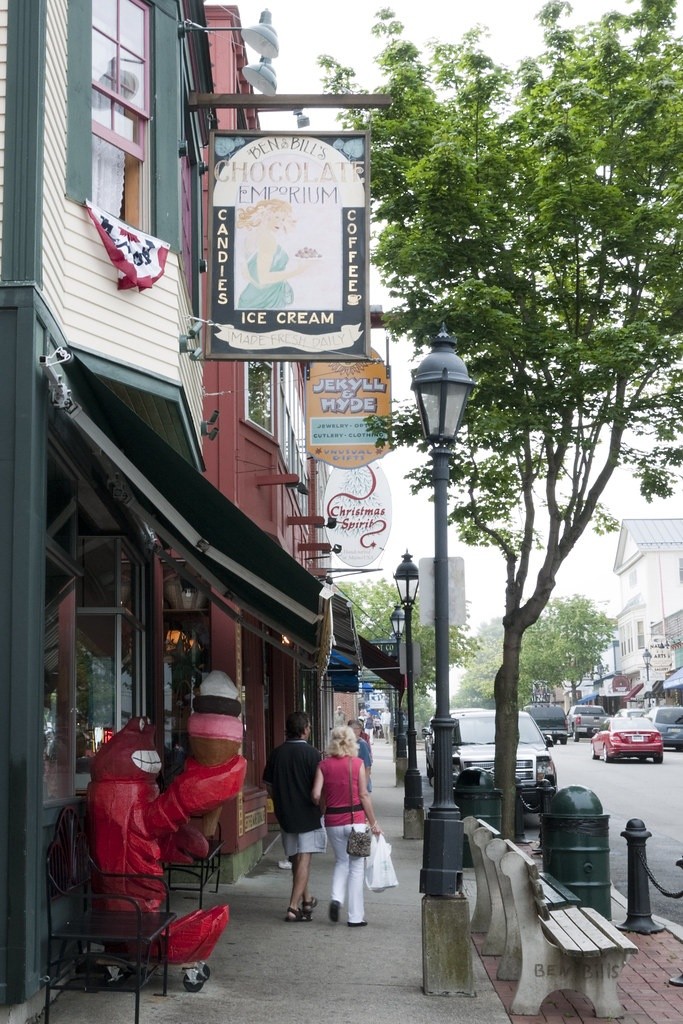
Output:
[161,813,225,910]
[44,805,178,1024]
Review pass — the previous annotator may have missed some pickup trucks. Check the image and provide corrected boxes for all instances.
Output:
[565,705,614,742]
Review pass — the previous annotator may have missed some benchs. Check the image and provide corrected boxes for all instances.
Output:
[463,815,581,955]
[484,838,639,1017]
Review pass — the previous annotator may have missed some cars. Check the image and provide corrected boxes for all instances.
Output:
[639,705,683,752]
[613,709,644,716]
[421,706,558,813]
[590,717,664,764]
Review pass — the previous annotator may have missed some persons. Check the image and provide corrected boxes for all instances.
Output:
[312,725,382,927]
[348,720,373,793]
[261,711,328,922]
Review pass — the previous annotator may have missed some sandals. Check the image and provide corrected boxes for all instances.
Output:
[285,907,313,921]
[302,896,318,913]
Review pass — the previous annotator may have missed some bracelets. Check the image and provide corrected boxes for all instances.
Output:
[371,821,377,829]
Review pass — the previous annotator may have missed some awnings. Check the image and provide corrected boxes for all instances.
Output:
[39,346,411,695]
[663,666,683,691]
[578,688,600,703]
[623,682,644,702]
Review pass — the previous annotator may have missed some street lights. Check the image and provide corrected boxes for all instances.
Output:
[642,649,652,707]
[391,548,426,808]
[388,600,408,757]
[411,319,476,891]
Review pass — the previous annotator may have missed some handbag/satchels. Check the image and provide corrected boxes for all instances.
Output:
[365,834,398,893]
[346,830,372,857]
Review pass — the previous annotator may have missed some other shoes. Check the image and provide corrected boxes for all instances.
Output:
[278,859,293,870]
[347,922,367,927]
[329,901,340,922]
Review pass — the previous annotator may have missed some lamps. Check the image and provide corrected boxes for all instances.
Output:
[201,409,219,441]
[318,576,333,585]
[179,321,203,361]
[322,544,342,554]
[285,482,310,496]
[293,108,310,128]
[314,517,337,529]
[93,57,144,110]
[186,19,278,97]
[178,8,280,59]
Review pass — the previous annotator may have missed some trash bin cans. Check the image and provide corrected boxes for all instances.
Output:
[454,766,503,867]
[539,786,612,921]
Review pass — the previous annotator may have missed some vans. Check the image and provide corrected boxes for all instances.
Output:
[523,703,569,745]
